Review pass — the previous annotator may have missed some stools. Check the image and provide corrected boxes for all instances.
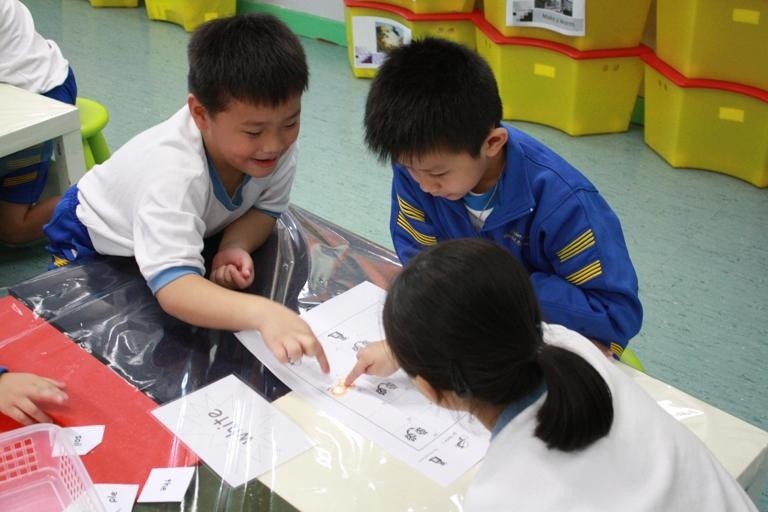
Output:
[54,97,112,172]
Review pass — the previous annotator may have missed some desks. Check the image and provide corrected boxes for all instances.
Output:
[1,205,767,512]
[0,81,87,199]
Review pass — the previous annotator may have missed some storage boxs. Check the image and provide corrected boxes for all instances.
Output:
[142,0,237,34]
[470,14,645,137]
[653,0,767,92]
[89,0,139,9]
[389,0,478,16]
[637,45,768,188]
[341,1,473,80]
[479,1,653,51]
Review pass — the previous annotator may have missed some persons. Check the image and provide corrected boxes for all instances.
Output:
[0,365,68,430]
[346,235,760,511]
[1,1,77,247]
[363,35,645,361]
[39,10,331,377]
